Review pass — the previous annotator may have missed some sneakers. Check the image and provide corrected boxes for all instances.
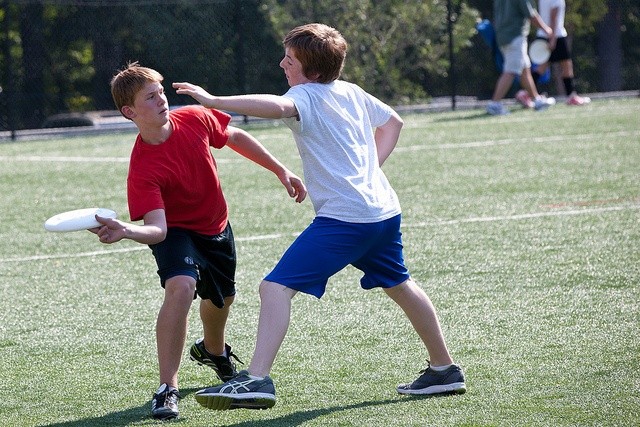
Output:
[515,90,535,108]
[487,102,510,115]
[566,95,584,105]
[195,370,276,411]
[190,338,244,383]
[535,95,556,111]
[396,360,466,395]
[151,383,184,420]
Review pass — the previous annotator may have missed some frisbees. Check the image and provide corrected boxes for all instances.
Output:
[44,209,116,233]
[528,40,550,65]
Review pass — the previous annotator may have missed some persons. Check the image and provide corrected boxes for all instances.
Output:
[87,59,308,420]
[486,0,557,116]
[172,23,468,409]
[514,0,592,109]
